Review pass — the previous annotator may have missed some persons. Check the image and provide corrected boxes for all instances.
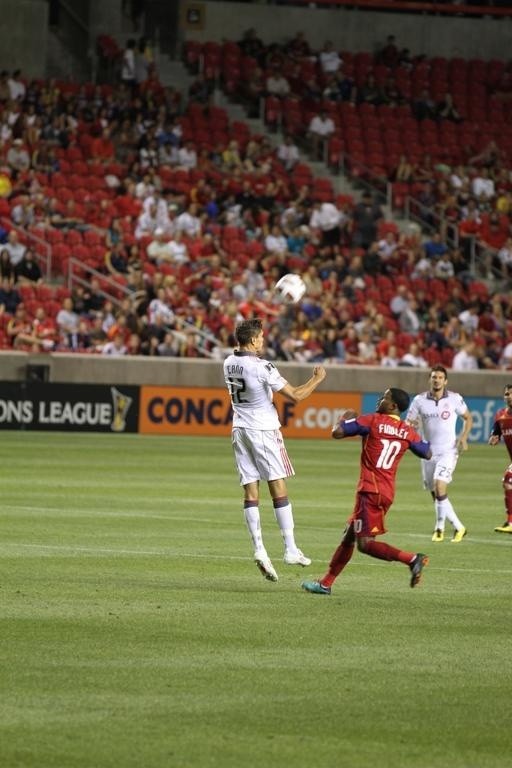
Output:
[223,318,327,583]
[486,383,512,534]
[300,387,432,596]
[1,25,512,373]
[404,366,472,544]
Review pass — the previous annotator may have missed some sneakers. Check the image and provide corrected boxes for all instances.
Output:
[450,528,467,542]
[411,553,429,588]
[494,522,512,534]
[284,549,312,566]
[254,552,278,581]
[432,528,444,541]
[301,582,331,595]
